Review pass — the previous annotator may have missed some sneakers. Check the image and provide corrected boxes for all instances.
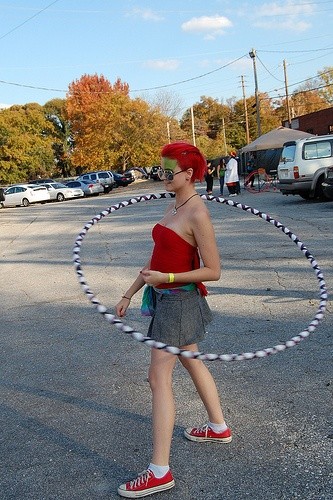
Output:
[207,190,212,194]
[118,468,175,498]
[183,424,232,444]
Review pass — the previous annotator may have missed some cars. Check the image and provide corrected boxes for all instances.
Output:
[65,180,104,196]
[37,183,84,201]
[112,173,135,188]
[0,184,50,207]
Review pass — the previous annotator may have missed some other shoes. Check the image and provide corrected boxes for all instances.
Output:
[228,193,236,197]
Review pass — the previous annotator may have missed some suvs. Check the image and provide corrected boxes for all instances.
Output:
[275,135,332,200]
[74,170,115,192]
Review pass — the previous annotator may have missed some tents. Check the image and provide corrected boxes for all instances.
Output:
[241,126,317,193]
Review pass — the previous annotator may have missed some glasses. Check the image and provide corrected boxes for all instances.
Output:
[157,168,189,180]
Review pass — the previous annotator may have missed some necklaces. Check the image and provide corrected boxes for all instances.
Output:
[171,194,199,215]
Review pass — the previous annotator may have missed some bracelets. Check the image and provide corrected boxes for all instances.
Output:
[122,296,131,300]
[169,273,175,283]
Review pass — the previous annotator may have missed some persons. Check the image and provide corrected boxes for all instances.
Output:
[244,155,256,187]
[204,154,241,198]
[117,144,233,498]
